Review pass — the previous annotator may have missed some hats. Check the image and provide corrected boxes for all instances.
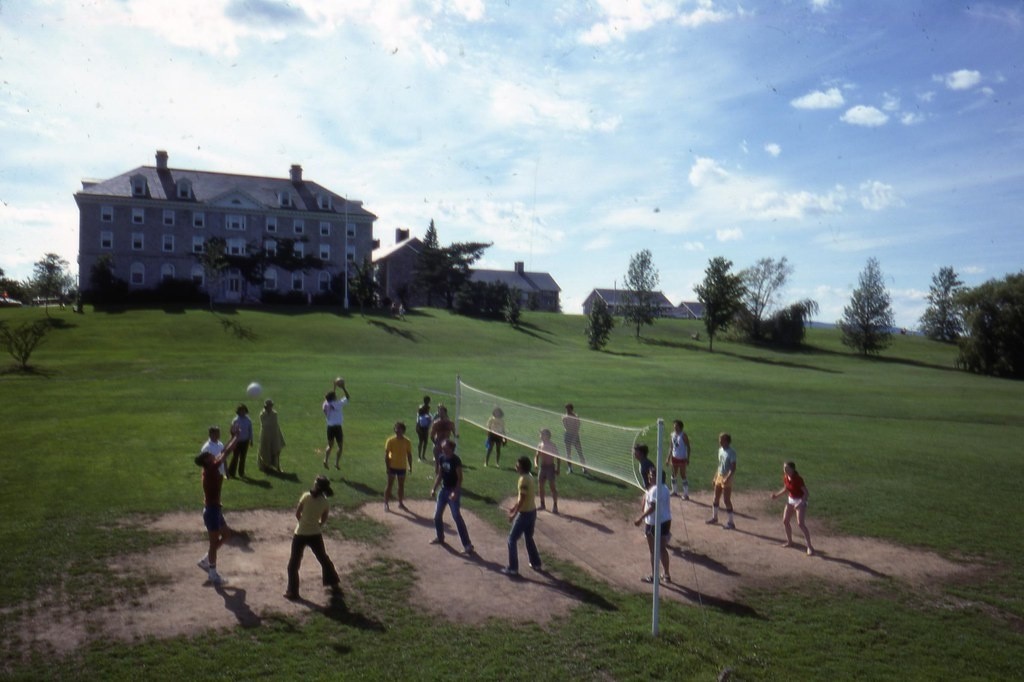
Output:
[265,400,274,406]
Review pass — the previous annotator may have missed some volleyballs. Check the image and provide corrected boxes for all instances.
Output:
[336,378,344,387]
[246,382,262,398]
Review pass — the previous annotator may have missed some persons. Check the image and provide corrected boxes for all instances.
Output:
[483,407,508,468]
[415,396,473,553]
[383,422,413,512]
[705,432,735,529]
[561,404,587,475]
[633,419,691,582]
[195,398,286,584]
[58,298,65,309]
[283,478,341,598]
[500,456,542,574]
[534,430,561,513]
[388,302,408,319]
[770,461,815,555]
[323,380,350,469]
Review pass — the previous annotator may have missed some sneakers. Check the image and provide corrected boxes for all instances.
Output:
[501,567,518,576]
[197,560,230,584]
[529,563,541,571]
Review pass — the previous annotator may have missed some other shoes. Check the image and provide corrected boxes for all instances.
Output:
[384,503,390,513]
[465,544,474,553]
[566,468,586,475]
[429,537,445,544]
[659,574,672,583]
[418,458,428,464]
[681,495,690,500]
[670,491,677,496]
[398,503,408,511]
[782,542,794,548]
[807,546,813,556]
[705,518,718,524]
[484,463,501,468]
[536,506,558,514]
[724,522,735,530]
[324,461,340,471]
[641,574,655,584]
